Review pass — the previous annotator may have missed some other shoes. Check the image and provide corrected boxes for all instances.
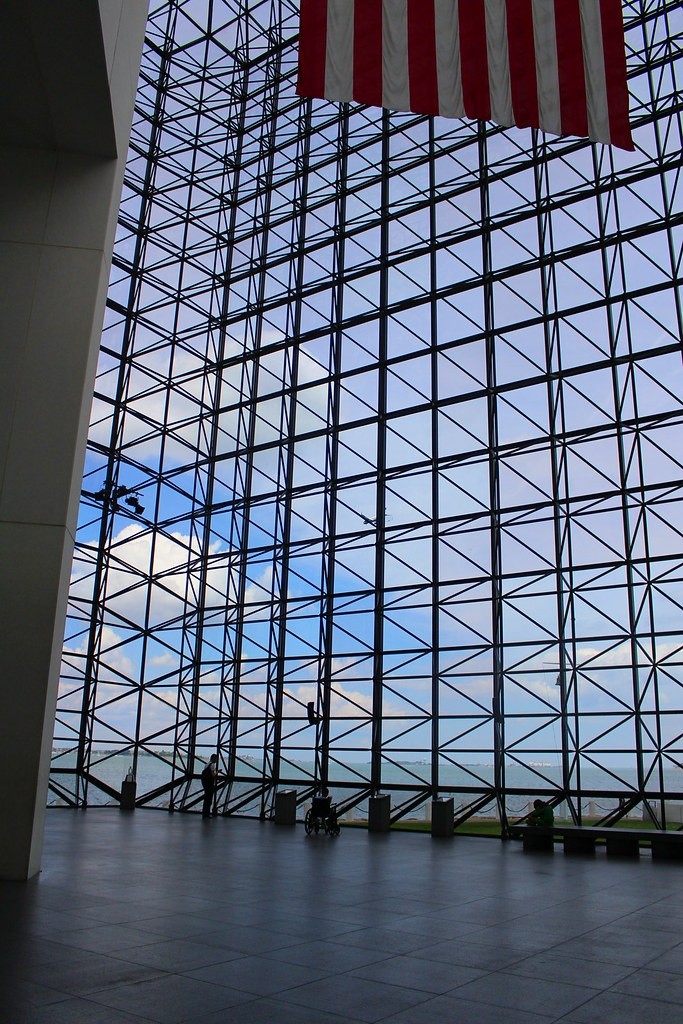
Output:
[202,816,213,820]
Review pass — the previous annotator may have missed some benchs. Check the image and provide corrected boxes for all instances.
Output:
[508,825,683,864]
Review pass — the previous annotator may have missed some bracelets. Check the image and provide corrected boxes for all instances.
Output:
[217,771,218,773]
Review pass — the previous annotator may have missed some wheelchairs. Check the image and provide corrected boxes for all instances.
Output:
[305,796,342,839]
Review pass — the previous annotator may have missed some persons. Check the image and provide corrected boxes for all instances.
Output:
[526,799,554,826]
[201,754,222,819]
[314,787,332,829]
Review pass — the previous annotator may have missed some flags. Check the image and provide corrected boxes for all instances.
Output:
[295,0,636,152]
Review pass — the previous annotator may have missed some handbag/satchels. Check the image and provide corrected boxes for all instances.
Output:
[201,762,215,790]
